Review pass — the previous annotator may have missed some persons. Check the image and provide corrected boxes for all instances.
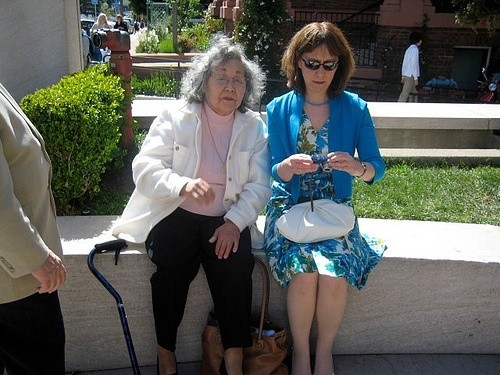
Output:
[113,33,270,374]
[397,31,422,102]
[88,13,112,63]
[0,78,69,375]
[133,17,148,34]
[265,20,386,375]
[112,14,129,34]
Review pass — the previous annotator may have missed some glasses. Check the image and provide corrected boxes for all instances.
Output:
[301,57,339,71]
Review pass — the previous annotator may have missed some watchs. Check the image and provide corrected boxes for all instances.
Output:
[357,161,367,178]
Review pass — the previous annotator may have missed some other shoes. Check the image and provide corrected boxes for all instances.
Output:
[219,357,245,375]
[156,351,178,375]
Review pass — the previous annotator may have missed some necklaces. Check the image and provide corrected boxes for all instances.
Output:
[202,105,234,169]
[305,99,329,106]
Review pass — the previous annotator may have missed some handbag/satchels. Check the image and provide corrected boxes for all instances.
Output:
[201,257,289,375]
[275,199,356,244]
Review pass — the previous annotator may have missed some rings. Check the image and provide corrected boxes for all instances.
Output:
[300,170,302,172]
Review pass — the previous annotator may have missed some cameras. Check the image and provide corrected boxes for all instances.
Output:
[310,153,334,173]
